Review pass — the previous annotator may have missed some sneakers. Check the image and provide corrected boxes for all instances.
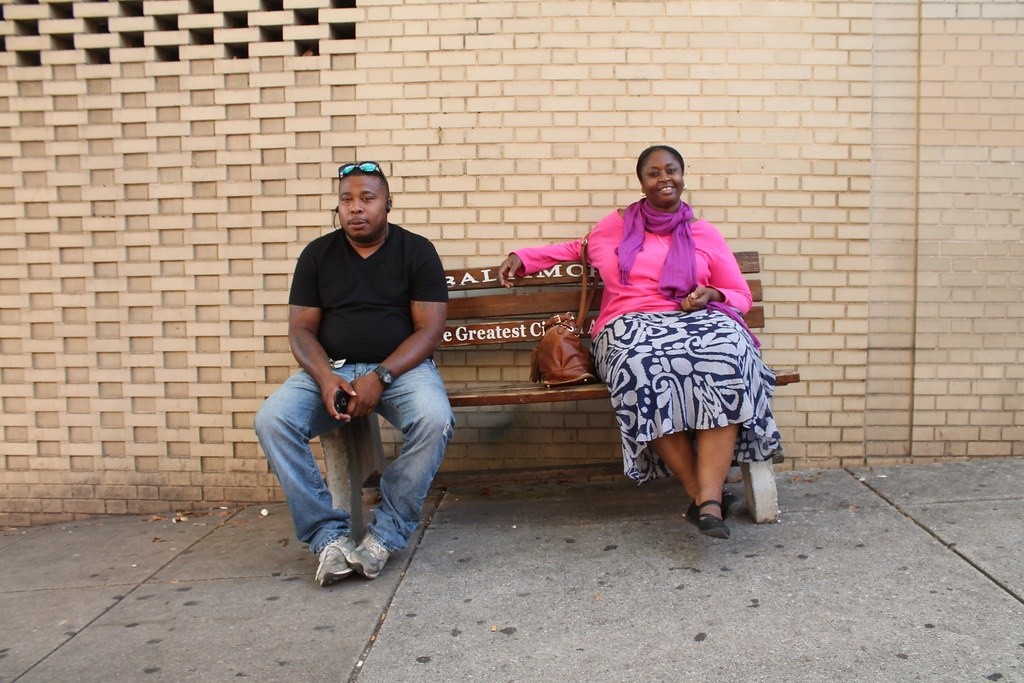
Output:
[315,535,357,587]
[347,531,391,580]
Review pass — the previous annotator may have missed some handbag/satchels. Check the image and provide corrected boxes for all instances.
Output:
[530,232,600,388]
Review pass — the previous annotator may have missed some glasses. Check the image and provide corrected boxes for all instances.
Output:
[339,161,384,179]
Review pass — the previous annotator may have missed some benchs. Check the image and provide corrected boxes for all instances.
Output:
[315,249,803,539]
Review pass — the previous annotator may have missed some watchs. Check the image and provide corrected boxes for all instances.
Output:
[373,365,394,389]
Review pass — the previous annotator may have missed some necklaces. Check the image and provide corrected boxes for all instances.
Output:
[657,234,669,252]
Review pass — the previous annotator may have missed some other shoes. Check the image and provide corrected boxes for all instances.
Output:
[686,497,730,538]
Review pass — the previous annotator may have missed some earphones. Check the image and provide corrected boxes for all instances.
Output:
[385,197,391,213]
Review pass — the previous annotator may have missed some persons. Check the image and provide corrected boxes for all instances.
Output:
[253,161,456,587]
[498,145,783,539]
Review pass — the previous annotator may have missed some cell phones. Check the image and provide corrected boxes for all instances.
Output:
[336,390,350,415]
